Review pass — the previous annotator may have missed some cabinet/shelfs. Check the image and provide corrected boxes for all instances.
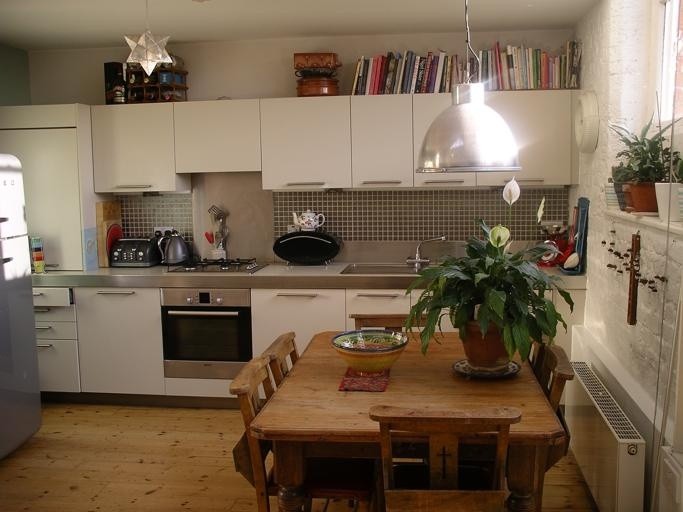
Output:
[248,286,344,399]
[91,103,192,193]
[341,287,586,368]
[413,94,476,191]
[32,287,81,395]
[0,104,119,272]
[73,284,165,396]
[476,91,576,190]
[175,99,261,172]
[351,95,413,192]
[126,67,188,104]
[261,97,351,190]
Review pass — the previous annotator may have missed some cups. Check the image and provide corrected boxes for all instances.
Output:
[31,237,47,273]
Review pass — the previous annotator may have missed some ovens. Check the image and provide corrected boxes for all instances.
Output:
[161,288,251,362]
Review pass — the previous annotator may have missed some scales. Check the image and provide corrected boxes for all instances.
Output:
[537,225,567,267]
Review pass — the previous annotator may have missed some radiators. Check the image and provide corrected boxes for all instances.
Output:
[565,362,646,512]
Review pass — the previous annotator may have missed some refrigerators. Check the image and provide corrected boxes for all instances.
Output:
[0,154,41,465]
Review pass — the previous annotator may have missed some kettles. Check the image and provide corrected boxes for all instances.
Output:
[157,229,189,266]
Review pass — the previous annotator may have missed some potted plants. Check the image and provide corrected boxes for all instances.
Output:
[653,146,683,222]
[607,162,633,211]
[607,113,683,216]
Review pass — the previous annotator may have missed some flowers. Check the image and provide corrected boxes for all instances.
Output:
[404,175,580,362]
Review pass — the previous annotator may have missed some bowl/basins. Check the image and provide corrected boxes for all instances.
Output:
[563,252,579,269]
[294,68,338,78]
[331,331,407,373]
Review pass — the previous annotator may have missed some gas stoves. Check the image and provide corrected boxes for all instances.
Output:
[169,256,268,272]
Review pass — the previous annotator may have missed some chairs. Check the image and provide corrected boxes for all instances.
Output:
[431,335,578,462]
[363,403,522,511]
[261,329,303,381]
[229,356,376,511]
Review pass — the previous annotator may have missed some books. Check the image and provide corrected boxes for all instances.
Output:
[351,40,583,95]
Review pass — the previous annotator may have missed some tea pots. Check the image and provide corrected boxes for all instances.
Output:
[291,208,325,233]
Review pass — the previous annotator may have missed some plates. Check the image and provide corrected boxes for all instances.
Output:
[452,359,522,381]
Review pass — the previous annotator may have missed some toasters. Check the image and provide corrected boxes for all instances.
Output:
[110,238,155,268]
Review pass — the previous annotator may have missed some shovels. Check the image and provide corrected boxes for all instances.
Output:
[207,205,225,249]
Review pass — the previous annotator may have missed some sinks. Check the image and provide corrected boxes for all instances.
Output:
[336,262,425,275]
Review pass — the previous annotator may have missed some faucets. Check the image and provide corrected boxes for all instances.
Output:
[406,235,447,267]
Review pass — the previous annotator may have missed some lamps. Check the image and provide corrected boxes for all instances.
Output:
[124,0,174,76]
[416,1,522,175]
